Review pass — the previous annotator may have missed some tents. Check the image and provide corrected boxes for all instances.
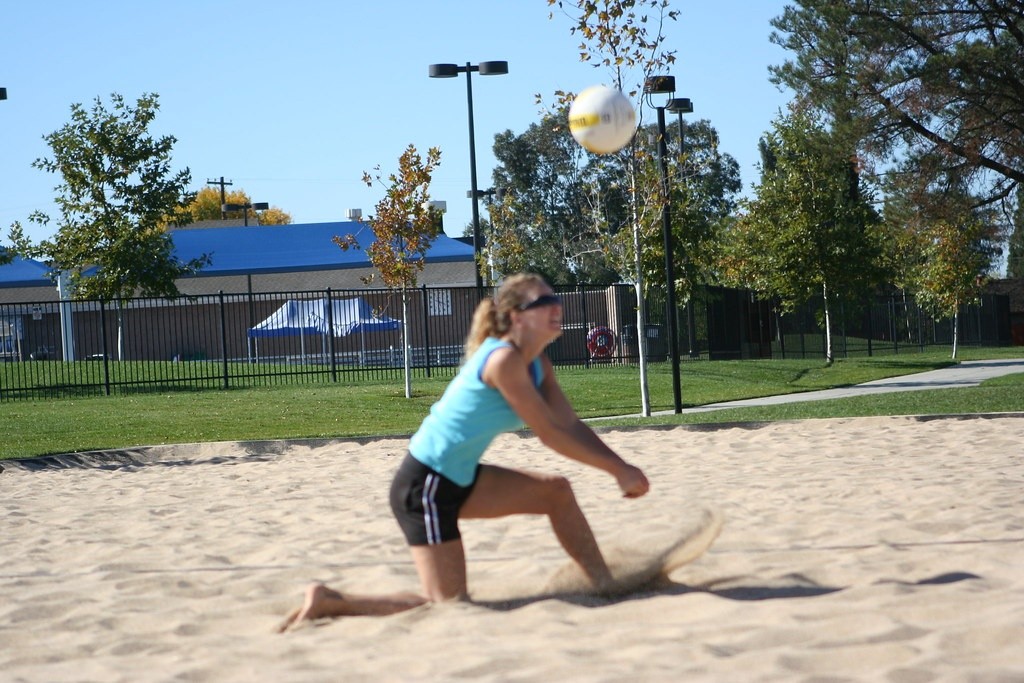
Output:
[246,297,404,365]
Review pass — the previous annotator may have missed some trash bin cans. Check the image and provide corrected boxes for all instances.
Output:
[626,321,666,360]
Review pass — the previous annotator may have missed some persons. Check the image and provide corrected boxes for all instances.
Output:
[285,270,650,632]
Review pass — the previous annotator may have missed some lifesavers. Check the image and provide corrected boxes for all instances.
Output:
[586,325,617,358]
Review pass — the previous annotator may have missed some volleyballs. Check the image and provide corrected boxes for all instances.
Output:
[567,84,639,155]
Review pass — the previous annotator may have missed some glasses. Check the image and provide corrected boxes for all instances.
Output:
[518,293,560,313]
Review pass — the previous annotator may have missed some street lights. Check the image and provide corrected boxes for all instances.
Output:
[427,60,510,304]
[665,97,696,359]
[642,76,683,413]
[221,202,270,359]
[466,188,508,240]
